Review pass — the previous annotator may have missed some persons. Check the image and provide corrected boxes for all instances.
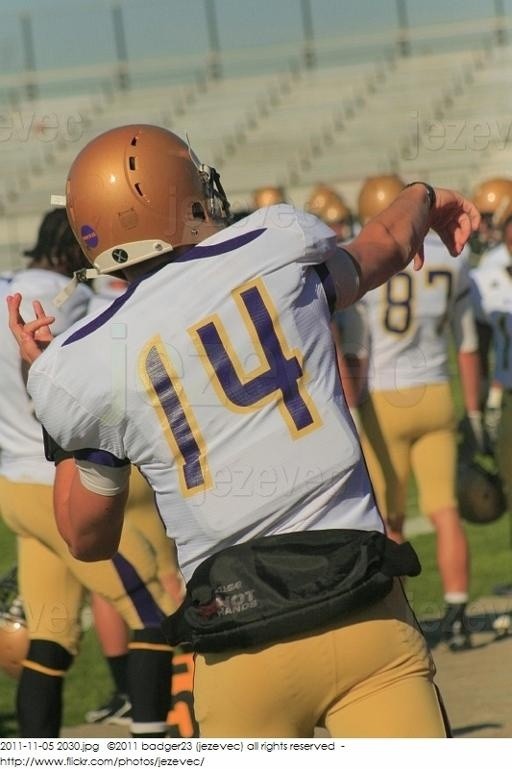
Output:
[0,122,512,738]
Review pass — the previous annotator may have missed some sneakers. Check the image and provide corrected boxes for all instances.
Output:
[85,694,134,724]
[446,606,470,651]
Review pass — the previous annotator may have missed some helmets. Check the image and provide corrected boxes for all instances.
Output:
[256,177,406,238]
[476,179,512,223]
[64,125,232,273]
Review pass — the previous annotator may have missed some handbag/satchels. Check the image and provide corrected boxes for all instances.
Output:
[162,528,422,650]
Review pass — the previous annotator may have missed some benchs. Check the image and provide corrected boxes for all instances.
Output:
[0,43,512,214]
